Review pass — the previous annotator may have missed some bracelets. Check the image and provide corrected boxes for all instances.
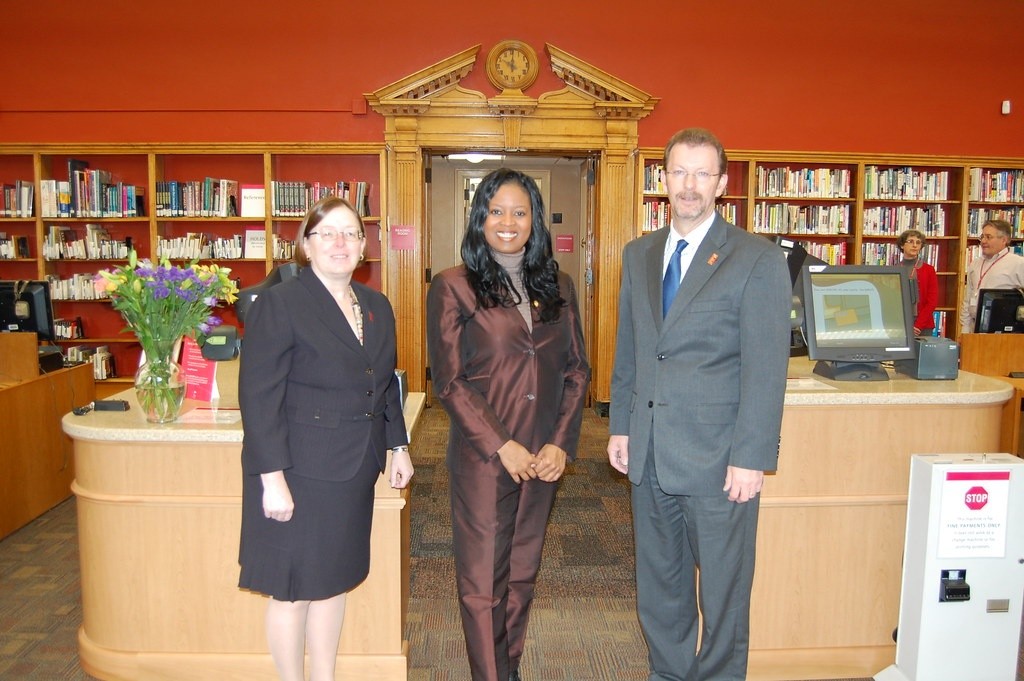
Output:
[392,447,409,453]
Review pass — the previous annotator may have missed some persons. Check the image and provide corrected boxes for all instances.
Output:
[893,230,938,337]
[957,218,1024,342]
[426,166,591,681]
[237,196,415,681]
[606,127,791,681]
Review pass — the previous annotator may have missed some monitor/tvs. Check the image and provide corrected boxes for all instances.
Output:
[0,280,56,343]
[772,235,808,287]
[800,264,916,381]
[974,288,1024,334]
[235,260,302,323]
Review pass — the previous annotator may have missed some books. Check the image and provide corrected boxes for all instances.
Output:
[44,224,135,261]
[642,163,737,233]
[751,165,851,266]
[0,232,30,259]
[157,226,266,260]
[139,316,218,403]
[964,166,1024,286]
[40,158,145,218]
[52,317,115,380]
[862,166,949,339]
[0,181,34,218]
[44,271,111,301]
[271,181,370,260]
[154,178,265,219]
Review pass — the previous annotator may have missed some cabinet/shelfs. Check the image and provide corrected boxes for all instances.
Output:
[628,144,1024,341]
[0,142,389,384]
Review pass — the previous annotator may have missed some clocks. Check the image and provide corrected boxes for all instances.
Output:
[485,39,538,92]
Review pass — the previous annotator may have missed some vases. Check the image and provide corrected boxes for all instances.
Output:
[136,335,188,428]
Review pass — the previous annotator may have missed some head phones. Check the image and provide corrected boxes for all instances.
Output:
[14,280,31,319]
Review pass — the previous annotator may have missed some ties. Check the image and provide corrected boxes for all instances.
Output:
[662,240,689,319]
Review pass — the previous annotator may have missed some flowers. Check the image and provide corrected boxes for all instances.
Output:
[97,241,240,421]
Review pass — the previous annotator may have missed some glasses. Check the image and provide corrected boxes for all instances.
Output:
[979,234,998,240]
[904,239,923,245]
[308,228,363,242]
[664,169,721,183]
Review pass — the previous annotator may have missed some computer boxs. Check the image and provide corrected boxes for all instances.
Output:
[38,346,63,374]
